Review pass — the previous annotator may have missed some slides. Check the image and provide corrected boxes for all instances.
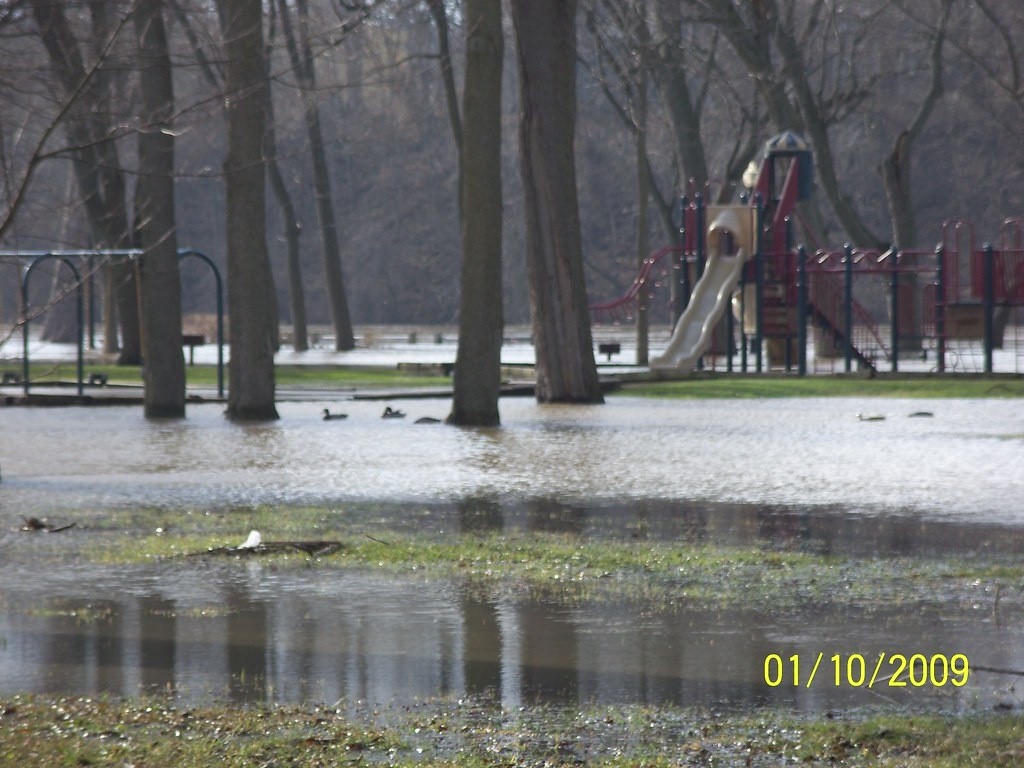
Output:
[648,247,747,373]
